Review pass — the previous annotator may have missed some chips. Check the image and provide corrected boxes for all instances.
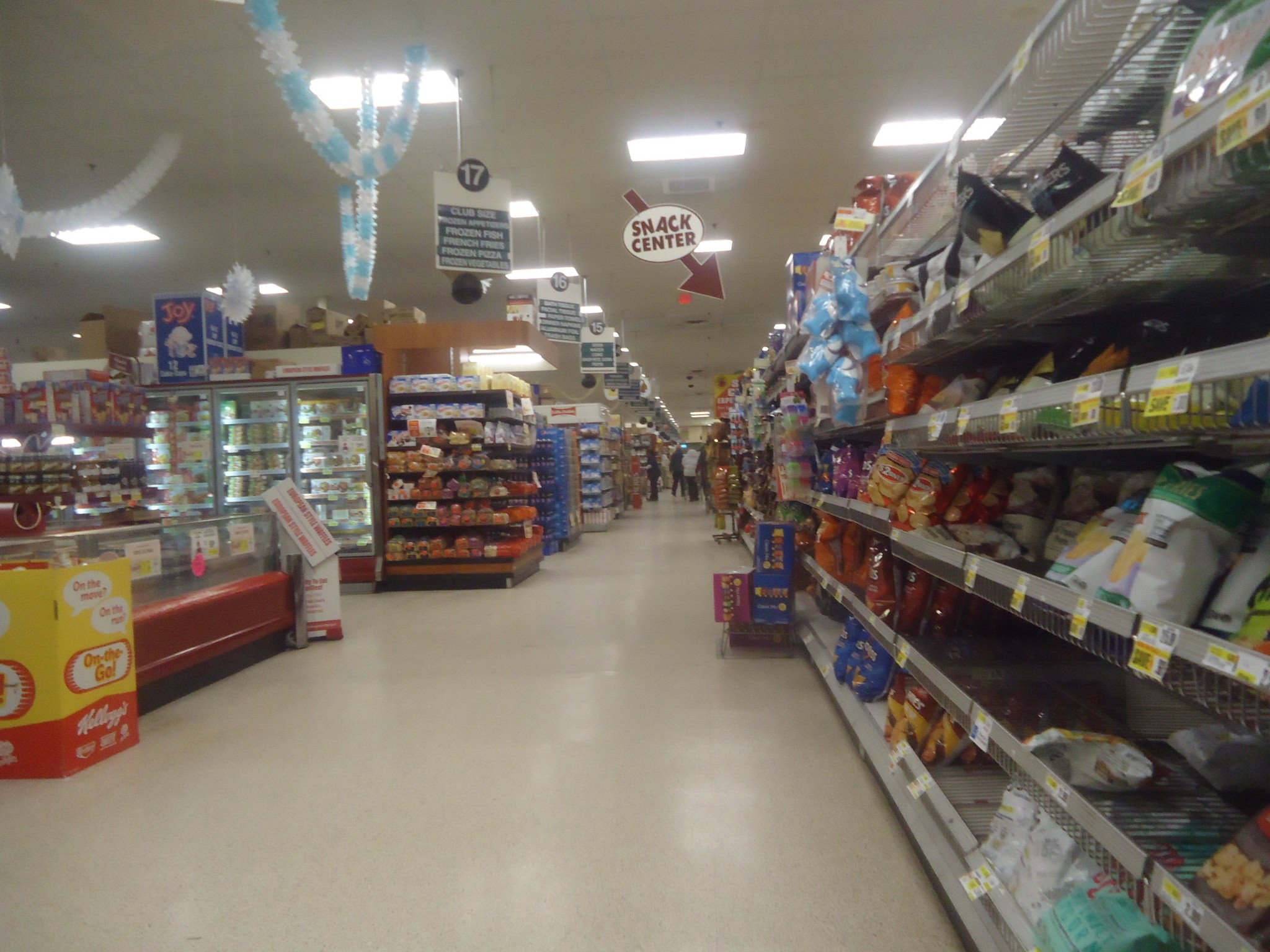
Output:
[1199,843,1270,909]
[1063,526,1151,583]
[866,470,1010,530]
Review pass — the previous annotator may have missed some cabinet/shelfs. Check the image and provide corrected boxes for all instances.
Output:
[0,423,156,504]
[574,424,664,531]
[737,1,1270,951]
[383,390,544,590]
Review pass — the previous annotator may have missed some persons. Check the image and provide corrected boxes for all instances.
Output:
[669,445,685,497]
[695,446,712,502]
[682,449,701,502]
[643,450,662,502]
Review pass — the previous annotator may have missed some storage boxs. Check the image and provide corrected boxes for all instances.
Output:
[80,289,427,358]
[713,520,796,624]
[385,373,478,446]
[0,348,16,396]
[781,253,818,330]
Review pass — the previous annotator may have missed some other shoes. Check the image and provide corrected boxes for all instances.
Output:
[649,497,657,500]
[690,497,698,502]
